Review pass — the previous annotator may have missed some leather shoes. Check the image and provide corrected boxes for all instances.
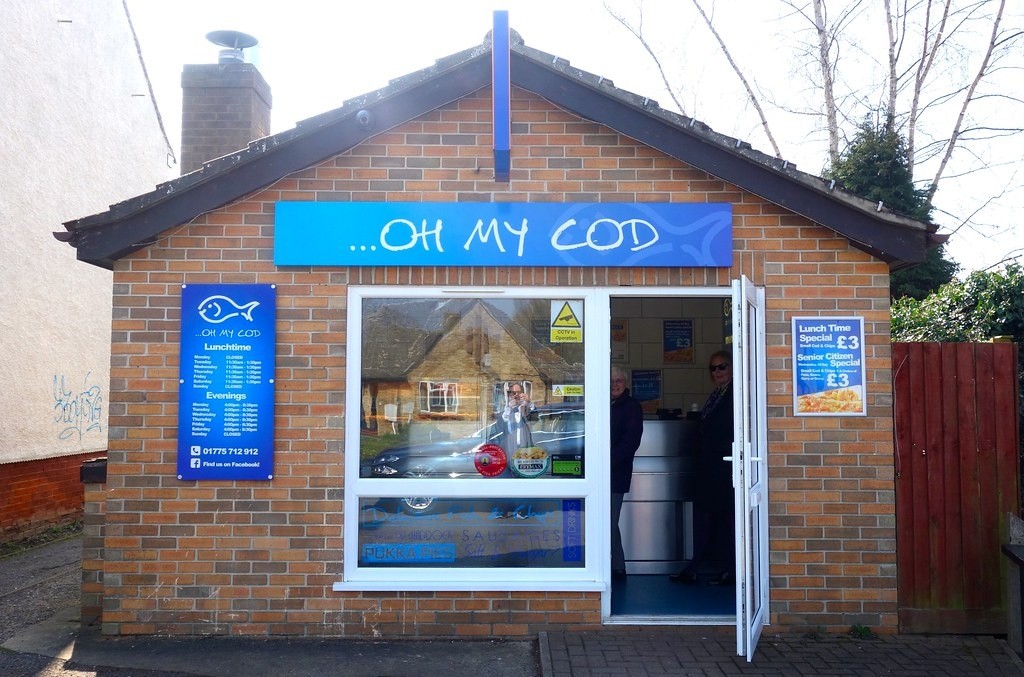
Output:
[669,571,698,585]
[706,577,724,585]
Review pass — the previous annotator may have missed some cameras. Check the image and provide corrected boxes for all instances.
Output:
[514,395,520,399]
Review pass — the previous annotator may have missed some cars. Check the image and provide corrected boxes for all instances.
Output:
[372,401,586,516]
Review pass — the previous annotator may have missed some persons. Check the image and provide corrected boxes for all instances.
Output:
[495,384,538,520]
[610,364,643,590]
[669,352,734,584]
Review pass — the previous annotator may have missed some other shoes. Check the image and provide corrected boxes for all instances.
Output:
[614,570,627,581]
[496,511,507,519]
[517,511,527,519]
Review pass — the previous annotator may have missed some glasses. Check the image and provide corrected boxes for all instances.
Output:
[709,363,730,372]
